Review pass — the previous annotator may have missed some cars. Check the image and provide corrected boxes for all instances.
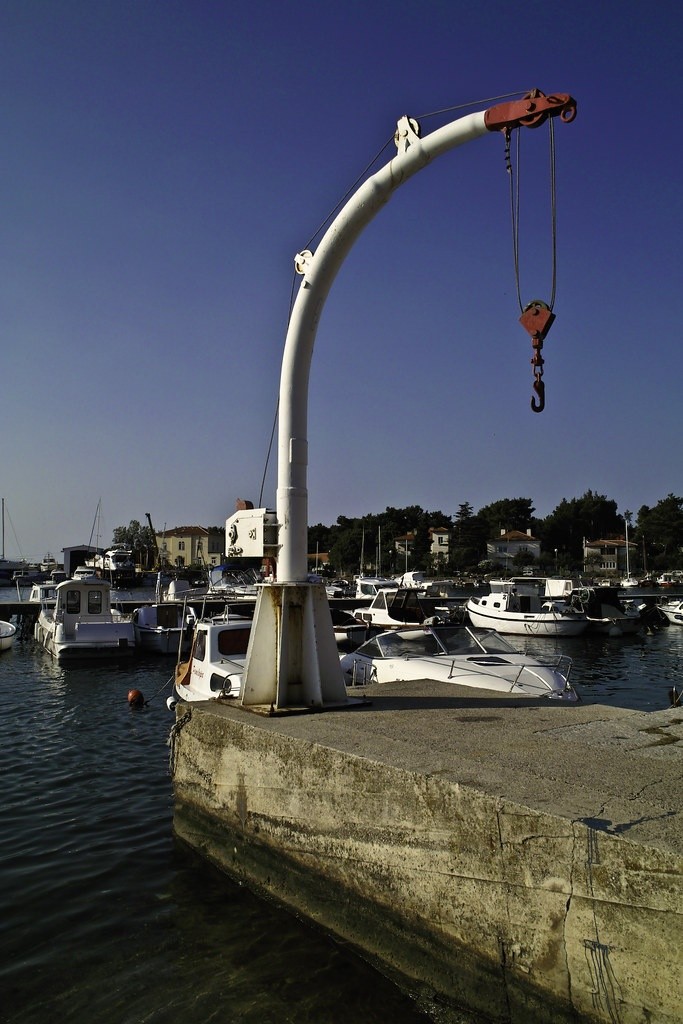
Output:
[657,601,683,625]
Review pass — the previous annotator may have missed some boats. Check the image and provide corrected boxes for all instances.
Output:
[136,602,191,655]
[352,586,457,641]
[331,607,366,643]
[163,520,683,599]
[327,608,582,703]
[12,570,31,588]
[27,581,59,602]
[172,605,254,702]
[97,548,138,586]
[0,619,16,650]
[33,576,138,659]
[467,581,588,635]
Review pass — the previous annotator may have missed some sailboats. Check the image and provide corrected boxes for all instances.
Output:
[69,494,103,580]
